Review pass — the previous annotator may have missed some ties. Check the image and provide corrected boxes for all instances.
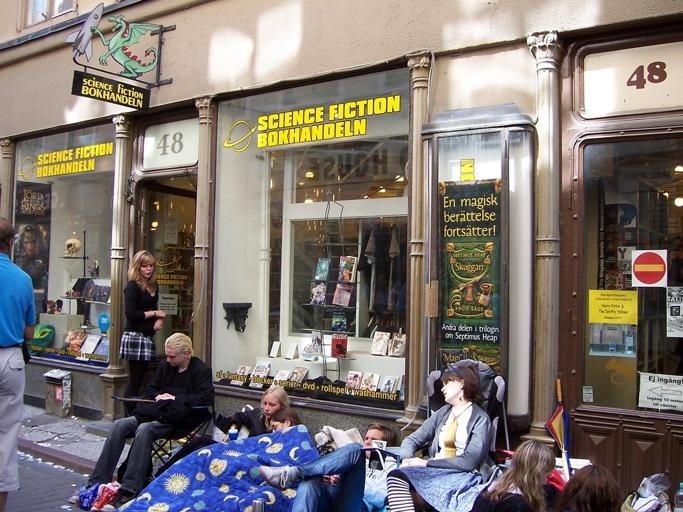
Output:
[445,417,460,460]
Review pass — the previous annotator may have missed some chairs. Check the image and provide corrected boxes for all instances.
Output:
[412,359,511,473]
[110,387,218,467]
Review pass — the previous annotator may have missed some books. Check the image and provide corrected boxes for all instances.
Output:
[269,255,360,361]
[227,361,402,400]
[369,327,407,357]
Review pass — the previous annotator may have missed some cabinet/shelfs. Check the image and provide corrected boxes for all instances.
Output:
[57,232,111,334]
[165,216,406,339]
[585,173,669,412]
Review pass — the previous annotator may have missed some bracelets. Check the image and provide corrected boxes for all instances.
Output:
[153,310,155,317]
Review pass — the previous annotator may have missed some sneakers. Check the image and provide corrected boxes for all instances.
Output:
[261,466,292,489]
[101,492,135,512]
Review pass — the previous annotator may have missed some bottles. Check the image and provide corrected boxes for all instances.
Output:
[674,481,683,512]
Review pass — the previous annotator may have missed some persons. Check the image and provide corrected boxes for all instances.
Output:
[117,248,166,418]
[258,424,396,512]
[0,216,36,512]
[478,439,555,512]
[222,386,289,442]
[268,409,300,433]
[68,332,214,511]
[548,464,624,512]
[386,365,492,512]
[13,224,48,291]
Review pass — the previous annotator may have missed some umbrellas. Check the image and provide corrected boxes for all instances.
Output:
[546,378,570,482]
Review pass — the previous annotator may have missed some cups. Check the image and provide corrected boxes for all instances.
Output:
[331,334,347,358]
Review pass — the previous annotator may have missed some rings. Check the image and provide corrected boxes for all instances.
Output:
[409,463,410,465]
